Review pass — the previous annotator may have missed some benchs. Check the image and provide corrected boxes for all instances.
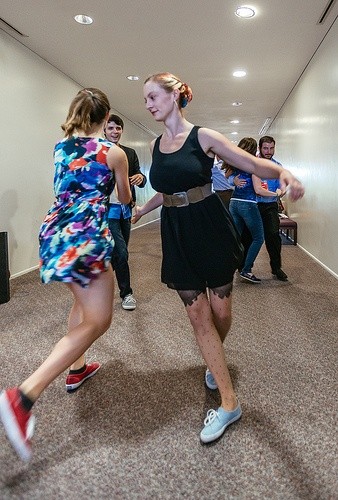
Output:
[279,213,297,246]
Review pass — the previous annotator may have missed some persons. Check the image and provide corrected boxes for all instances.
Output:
[212,136,289,283]
[103,114,147,309]
[0,87,130,464]
[132,73,305,442]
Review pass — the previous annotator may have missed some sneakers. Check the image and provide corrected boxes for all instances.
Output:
[0,386,35,461]
[66,362,101,389]
[240,271,261,284]
[271,269,288,280]
[121,294,136,309]
[199,398,242,443]
[206,366,220,389]
